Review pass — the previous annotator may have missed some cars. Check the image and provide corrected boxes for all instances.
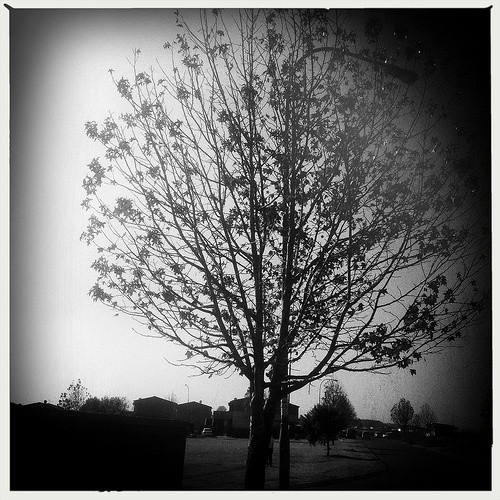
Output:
[346,423,460,449]
[202,428,213,436]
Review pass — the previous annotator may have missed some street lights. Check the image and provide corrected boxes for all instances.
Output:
[319,379,339,406]
[185,384,190,402]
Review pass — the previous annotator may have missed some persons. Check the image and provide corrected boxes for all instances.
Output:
[307,433,342,449]
[264,435,274,467]
